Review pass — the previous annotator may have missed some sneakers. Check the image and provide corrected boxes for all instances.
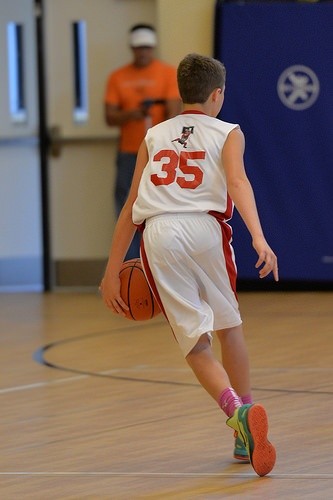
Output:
[225,403,275,477]
[234,432,250,460]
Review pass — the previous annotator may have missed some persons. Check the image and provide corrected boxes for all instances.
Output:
[105,23,183,264]
[98,53,280,476]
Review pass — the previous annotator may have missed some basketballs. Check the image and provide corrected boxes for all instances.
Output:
[113,255,163,320]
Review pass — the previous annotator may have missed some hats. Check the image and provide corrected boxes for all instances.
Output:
[129,27,156,47]
